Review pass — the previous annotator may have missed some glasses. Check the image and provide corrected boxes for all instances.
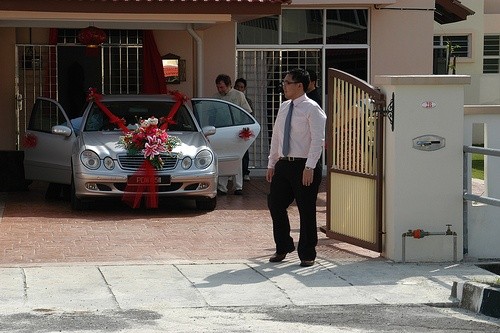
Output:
[282,80,300,86]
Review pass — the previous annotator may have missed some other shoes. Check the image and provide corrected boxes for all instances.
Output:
[51,196,64,201]
[229,176,232,181]
[299,260,314,266]
[217,189,226,196]
[234,190,244,195]
[270,245,295,262]
[244,174,250,181]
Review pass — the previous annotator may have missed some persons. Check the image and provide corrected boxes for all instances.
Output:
[264,68,327,266]
[207,73,253,195]
[45,113,114,202]
[234,77,255,181]
[305,70,323,189]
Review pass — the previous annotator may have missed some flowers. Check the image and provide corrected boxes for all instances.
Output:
[117,114,182,162]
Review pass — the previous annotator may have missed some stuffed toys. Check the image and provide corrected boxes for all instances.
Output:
[139,117,159,134]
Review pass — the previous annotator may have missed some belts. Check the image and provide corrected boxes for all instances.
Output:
[280,157,301,161]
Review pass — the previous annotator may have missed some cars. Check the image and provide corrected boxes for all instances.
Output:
[23,94,261,211]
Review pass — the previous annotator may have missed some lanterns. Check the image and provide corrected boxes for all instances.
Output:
[78,26,106,57]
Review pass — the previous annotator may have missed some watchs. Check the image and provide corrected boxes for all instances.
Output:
[305,167,311,170]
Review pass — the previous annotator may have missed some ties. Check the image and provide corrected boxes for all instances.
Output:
[282,101,294,157]
